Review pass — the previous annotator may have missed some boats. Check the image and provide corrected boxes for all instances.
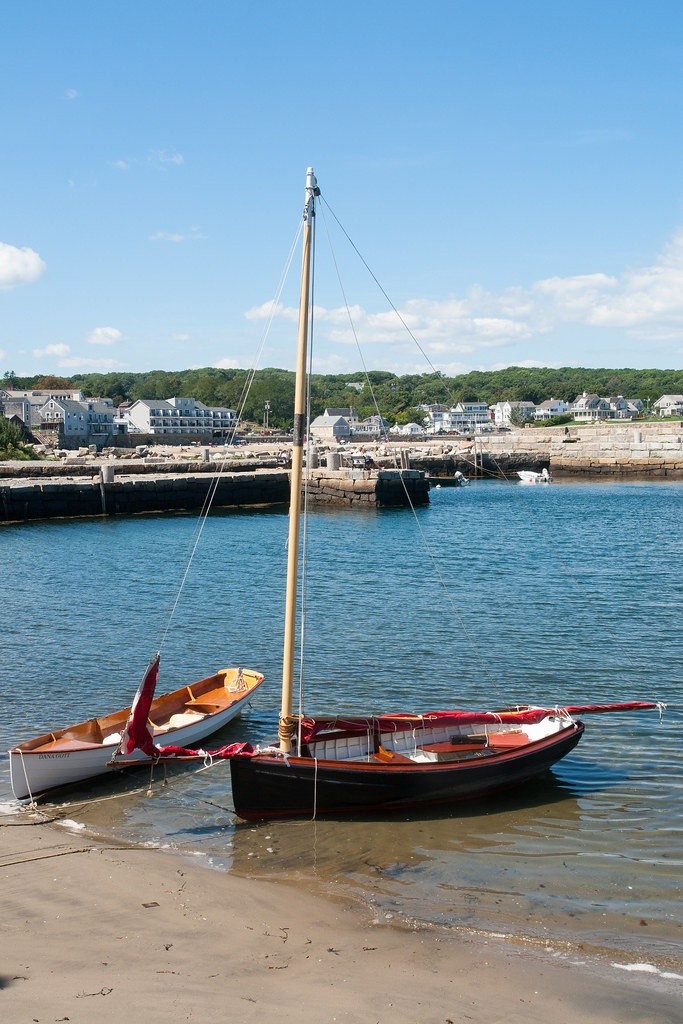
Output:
[7,664,267,803]
[515,467,554,484]
[425,470,471,488]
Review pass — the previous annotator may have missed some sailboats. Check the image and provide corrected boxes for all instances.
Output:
[108,164,682,830]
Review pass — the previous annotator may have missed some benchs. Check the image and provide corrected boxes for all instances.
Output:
[366,749,416,763]
